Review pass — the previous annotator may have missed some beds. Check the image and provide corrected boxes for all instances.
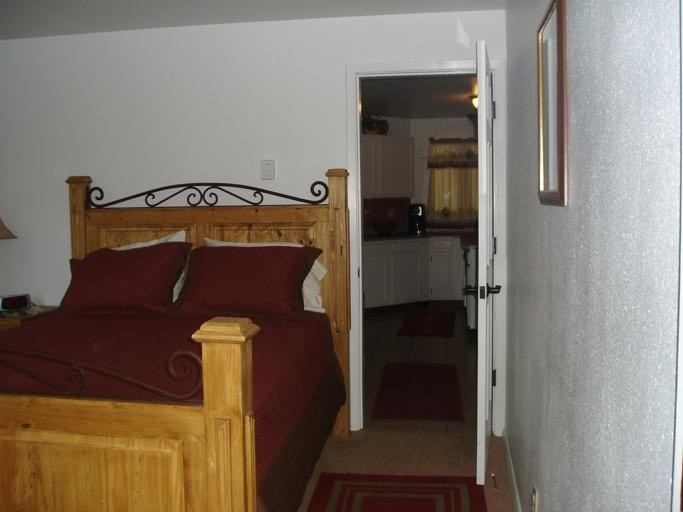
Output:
[1,168,350,512]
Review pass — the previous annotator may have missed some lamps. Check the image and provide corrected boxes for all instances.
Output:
[468,79,478,109]
[0,218,18,240]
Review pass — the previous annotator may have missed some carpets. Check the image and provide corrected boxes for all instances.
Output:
[306,472,488,511]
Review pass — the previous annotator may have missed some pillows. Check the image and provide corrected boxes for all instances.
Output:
[59,242,192,315]
[174,245,322,314]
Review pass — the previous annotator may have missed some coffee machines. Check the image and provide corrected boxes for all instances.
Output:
[407,202,425,238]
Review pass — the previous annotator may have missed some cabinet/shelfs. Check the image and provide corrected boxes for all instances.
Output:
[361,134,415,200]
[427,235,468,302]
[463,244,476,330]
[364,237,428,310]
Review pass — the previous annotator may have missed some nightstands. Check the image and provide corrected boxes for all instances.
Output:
[1,303,60,330]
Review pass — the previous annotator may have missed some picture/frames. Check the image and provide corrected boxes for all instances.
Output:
[536,1,568,207]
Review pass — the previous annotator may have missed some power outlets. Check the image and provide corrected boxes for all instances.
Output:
[530,484,539,512]
